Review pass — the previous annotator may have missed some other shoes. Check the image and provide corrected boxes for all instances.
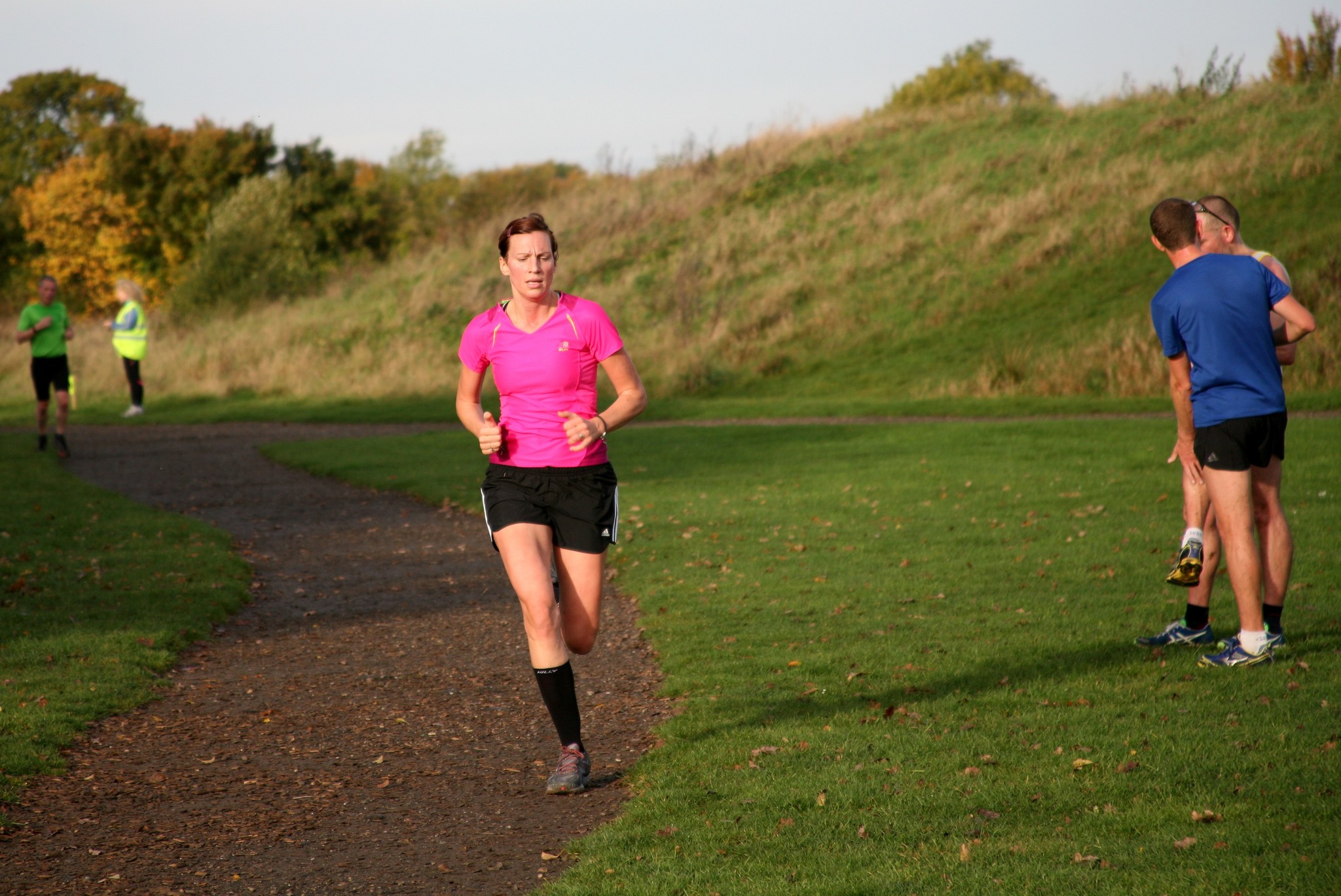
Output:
[124,406,141,417]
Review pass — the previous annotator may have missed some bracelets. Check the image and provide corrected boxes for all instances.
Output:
[594,415,607,440]
[32,327,37,334]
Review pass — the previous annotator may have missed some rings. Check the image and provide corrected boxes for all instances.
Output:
[578,433,583,439]
[489,442,493,451]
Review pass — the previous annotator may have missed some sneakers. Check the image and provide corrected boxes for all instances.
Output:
[1216,623,1286,653]
[1197,633,1273,670]
[52,435,69,457]
[1167,540,1203,587]
[547,746,591,794]
[1132,619,1215,648]
[37,433,48,451]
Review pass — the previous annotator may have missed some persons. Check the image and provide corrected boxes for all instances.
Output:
[16,275,72,459]
[455,214,647,792]
[102,279,148,419]
[1147,196,1317,673]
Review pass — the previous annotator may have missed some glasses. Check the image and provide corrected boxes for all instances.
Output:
[1192,201,1229,227]
